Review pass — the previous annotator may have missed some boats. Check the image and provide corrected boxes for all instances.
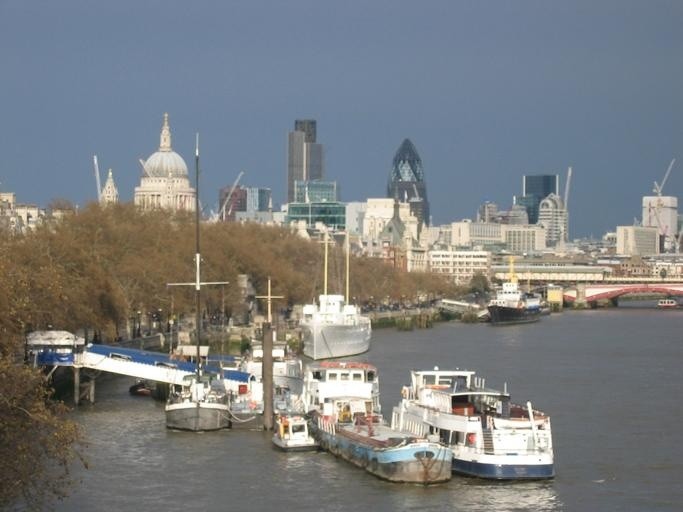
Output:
[657,297,682,310]
